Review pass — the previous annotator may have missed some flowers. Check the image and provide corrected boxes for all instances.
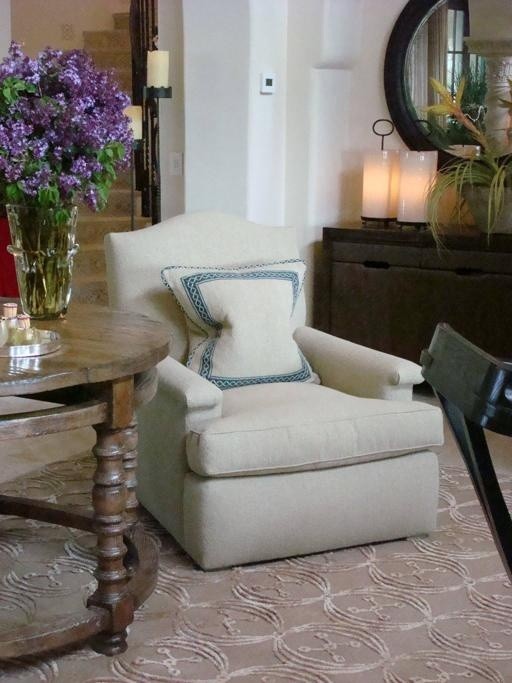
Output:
[0,40,133,315]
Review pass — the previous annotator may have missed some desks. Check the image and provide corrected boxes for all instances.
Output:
[0,297,171,661]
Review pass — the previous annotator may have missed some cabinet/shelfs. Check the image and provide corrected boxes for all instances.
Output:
[323,226,512,395]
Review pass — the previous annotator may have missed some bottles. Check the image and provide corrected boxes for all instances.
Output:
[0,302,40,345]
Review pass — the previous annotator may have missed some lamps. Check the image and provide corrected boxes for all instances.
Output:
[361,119,397,229]
[124,106,142,230]
[447,120,480,159]
[146,51,172,223]
[398,119,438,231]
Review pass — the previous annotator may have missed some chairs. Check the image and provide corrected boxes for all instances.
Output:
[420,323,512,584]
[104,211,445,571]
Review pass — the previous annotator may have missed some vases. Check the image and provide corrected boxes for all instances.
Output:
[5,204,80,322]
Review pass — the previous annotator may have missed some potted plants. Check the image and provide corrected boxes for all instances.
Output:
[424,76,512,261]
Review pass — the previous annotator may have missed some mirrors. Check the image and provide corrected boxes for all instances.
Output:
[383,0,512,177]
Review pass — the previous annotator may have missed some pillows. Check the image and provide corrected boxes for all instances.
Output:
[161,258,318,387]
